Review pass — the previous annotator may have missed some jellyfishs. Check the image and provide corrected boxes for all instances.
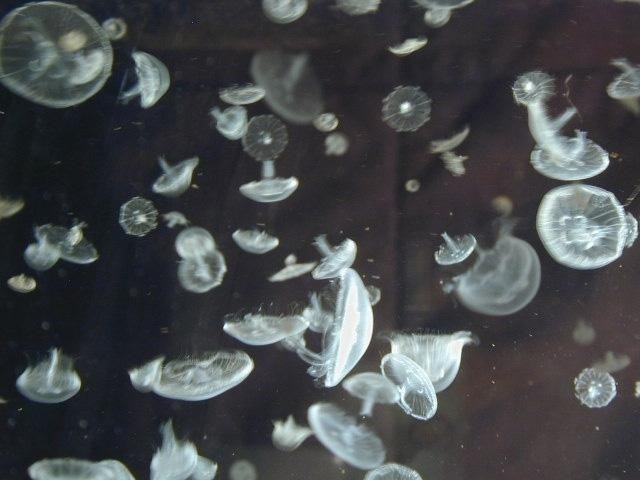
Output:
[0,0,640,480]
